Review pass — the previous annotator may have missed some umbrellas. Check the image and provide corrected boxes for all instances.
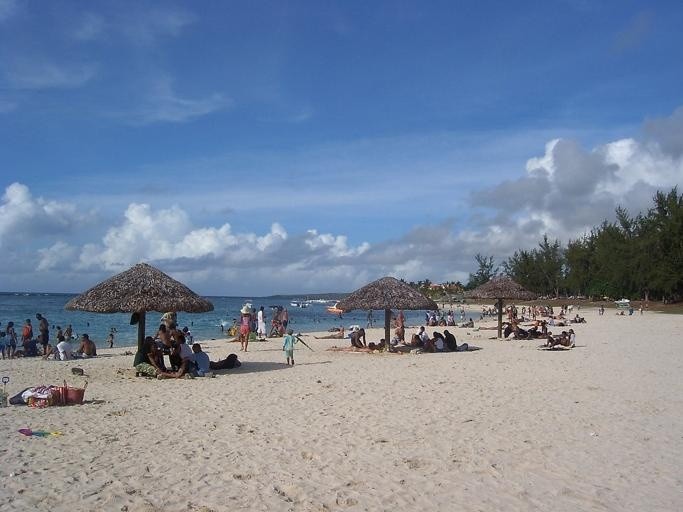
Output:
[335,276,439,350]
[463,275,537,338]
[65,262,215,352]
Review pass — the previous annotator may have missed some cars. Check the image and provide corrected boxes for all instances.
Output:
[615,298,630,306]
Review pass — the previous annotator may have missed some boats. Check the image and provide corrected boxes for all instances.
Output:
[290,299,309,307]
[326,307,344,314]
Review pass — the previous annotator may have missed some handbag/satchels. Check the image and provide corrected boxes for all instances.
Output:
[62,377,88,405]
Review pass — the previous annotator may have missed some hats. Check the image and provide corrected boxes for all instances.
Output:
[239,305,250,314]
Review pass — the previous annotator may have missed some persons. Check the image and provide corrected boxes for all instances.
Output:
[133,312,212,381]
[106,334,114,348]
[113,327,118,333]
[110,328,113,333]
[313,303,586,353]
[283,329,298,365]
[0,313,96,361]
[221,301,288,352]
[210,354,241,369]
[598,296,649,317]
